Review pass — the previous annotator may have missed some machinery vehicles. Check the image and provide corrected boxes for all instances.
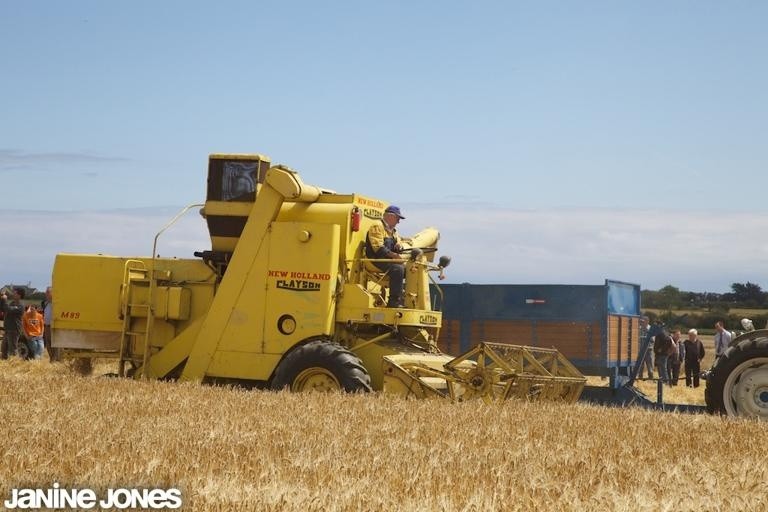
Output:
[427,279,767,417]
[51,154,588,403]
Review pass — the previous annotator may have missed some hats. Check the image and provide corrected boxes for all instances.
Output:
[385,206,406,219]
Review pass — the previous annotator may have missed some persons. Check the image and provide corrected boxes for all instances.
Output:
[682,328,707,389]
[668,330,687,388]
[0,285,24,360]
[365,204,407,311]
[43,287,62,363]
[713,320,733,358]
[21,304,45,359]
[652,320,677,386]
[635,314,655,380]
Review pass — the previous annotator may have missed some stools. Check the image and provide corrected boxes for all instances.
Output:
[362,255,383,274]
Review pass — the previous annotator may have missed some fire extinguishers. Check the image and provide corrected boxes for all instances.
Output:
[351,207,359,231]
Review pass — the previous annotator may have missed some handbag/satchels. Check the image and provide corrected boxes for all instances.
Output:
[663,335,677,356]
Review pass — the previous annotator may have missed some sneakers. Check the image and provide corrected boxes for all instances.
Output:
[386,301,408,308]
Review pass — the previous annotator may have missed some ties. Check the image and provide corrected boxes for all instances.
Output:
[718,332,723,353]
